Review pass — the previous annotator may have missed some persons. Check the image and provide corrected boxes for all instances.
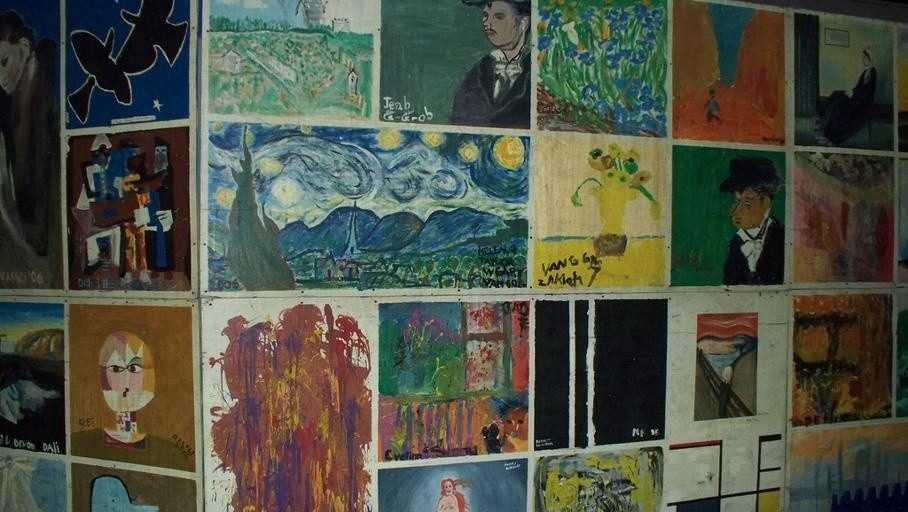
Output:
[716,155,786,286]
[447,0,532,131]
[1,6,62,286]
[699,86,723,123]
[435,478,460,512]
[811,44,878,148]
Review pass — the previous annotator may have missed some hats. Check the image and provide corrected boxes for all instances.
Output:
[719,156,781,194]
